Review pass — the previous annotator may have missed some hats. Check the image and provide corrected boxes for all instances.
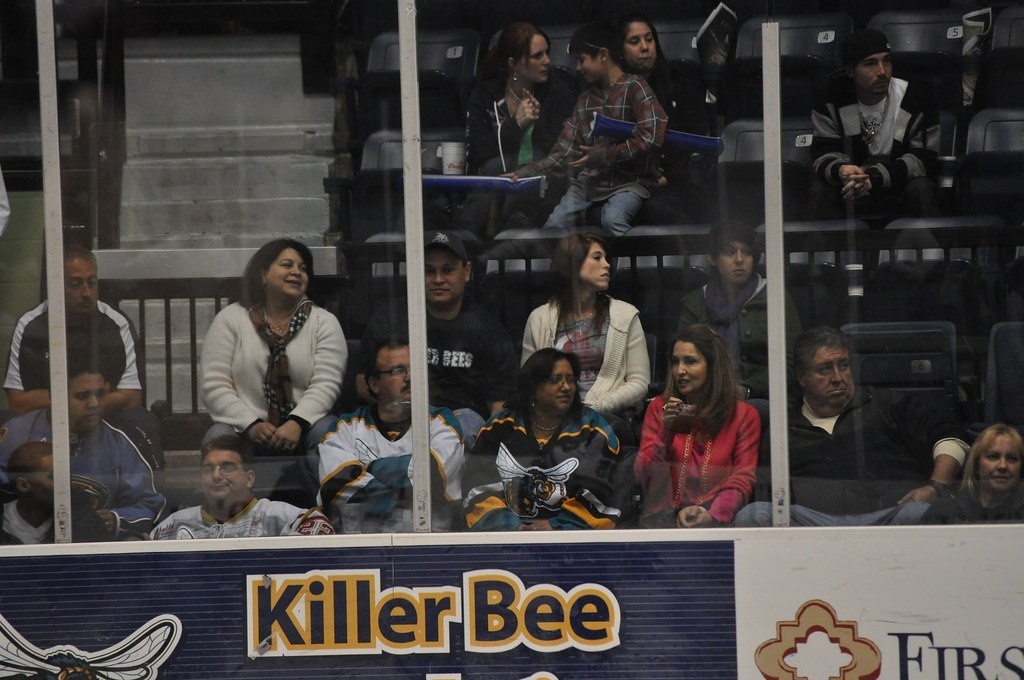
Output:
[423,230,468,267]
[841,27,891,67]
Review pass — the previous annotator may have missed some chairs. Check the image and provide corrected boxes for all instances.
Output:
[1,1,1023,524]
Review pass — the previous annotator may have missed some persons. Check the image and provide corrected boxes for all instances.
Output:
[355,229,513,445]
[515,233,653,415]
[467,13,942,252]
[678,216,799,400]
[638,324,761,529]
[462,347,637,532]
[734,327,1023,526]
[0,237,349,541]
[0,166,10,240]
[315,336,468,534]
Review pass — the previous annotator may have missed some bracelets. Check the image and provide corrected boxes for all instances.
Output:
[747,387,750,399]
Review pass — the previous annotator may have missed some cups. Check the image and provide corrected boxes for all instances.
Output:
[441,142,468,175]
[937,155,955,188]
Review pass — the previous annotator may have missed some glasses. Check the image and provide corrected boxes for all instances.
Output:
[199,463,244,476]
[544,375,581,388]
[374,366,407,378]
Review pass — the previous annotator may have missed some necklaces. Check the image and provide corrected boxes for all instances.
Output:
[507,84,534,102]
[861,95,890,135]
[562,296,600,341]
[533,417,562,431]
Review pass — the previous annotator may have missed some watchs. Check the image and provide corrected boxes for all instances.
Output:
[927,478,950,498]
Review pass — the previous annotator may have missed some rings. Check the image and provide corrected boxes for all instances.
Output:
[662,404,667,410]
[283,446,290,451]
[106,521,109,527]
